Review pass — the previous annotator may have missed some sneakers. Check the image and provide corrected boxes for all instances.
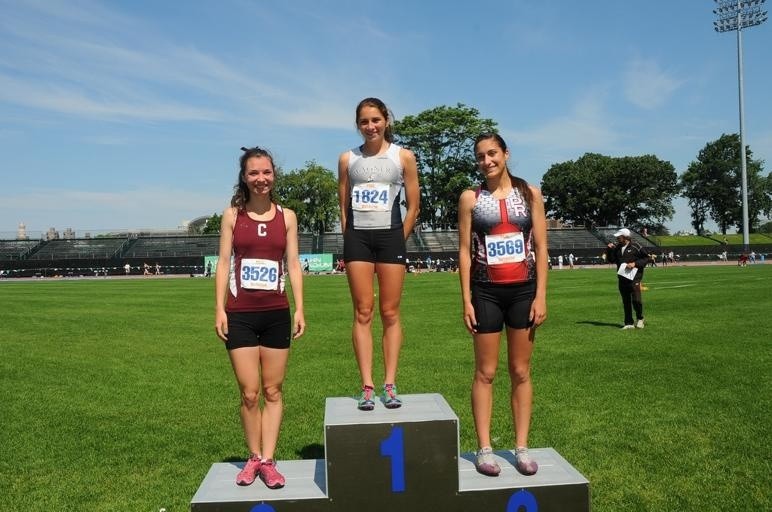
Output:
[259,458,286,488]
[357,384,376,410]
[474,447,501,476]
[622,323,635,329]
[235,453,261,485]
[636,318,645,328]
[380,383,401,409]
[514,447,538,475]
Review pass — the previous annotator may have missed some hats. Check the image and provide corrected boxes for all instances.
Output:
[613,228,631,238]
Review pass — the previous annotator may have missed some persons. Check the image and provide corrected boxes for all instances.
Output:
[208,261,212,279]
[335,257,344,272]
[142,262,165,279]
[647,249,680,267]
[599,251,609,265]
[547,253,574,270]
[304,259,310,272]
[335,98,421,409]
[214,144,308,490]
[406,254,456,272]
[124,262,133,277]
[721,249,757,268]
[607,228,647,329]
[456,132,550,478]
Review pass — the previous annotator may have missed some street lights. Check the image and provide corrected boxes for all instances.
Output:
[713,0,767,253]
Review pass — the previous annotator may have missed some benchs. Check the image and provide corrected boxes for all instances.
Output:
[2,225,657,259]
